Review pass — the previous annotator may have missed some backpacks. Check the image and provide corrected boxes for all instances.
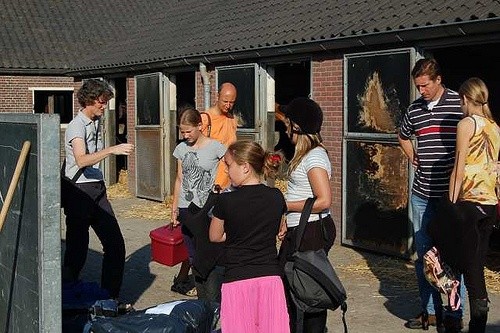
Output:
[283,196,347,314]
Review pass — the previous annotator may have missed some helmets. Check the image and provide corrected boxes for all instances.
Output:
[278,97,323,134]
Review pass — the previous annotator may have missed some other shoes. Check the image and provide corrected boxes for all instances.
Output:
[408,311,437,330]
[171,277,197,296]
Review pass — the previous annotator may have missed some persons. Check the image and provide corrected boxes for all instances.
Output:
[171,82,237,296]
[397,58,465,329]
[209,140,291,333]
[439,78,500,333]
[171,108,231,298]
[278,98,336,333]
[61,79,134,314]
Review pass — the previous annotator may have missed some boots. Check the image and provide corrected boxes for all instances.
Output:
[466,298,490,333]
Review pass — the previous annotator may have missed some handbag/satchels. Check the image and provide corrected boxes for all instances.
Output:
[61,167,95,222]
[423,247,463,311]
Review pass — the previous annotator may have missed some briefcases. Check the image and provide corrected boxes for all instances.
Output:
[149,220,189,266]
